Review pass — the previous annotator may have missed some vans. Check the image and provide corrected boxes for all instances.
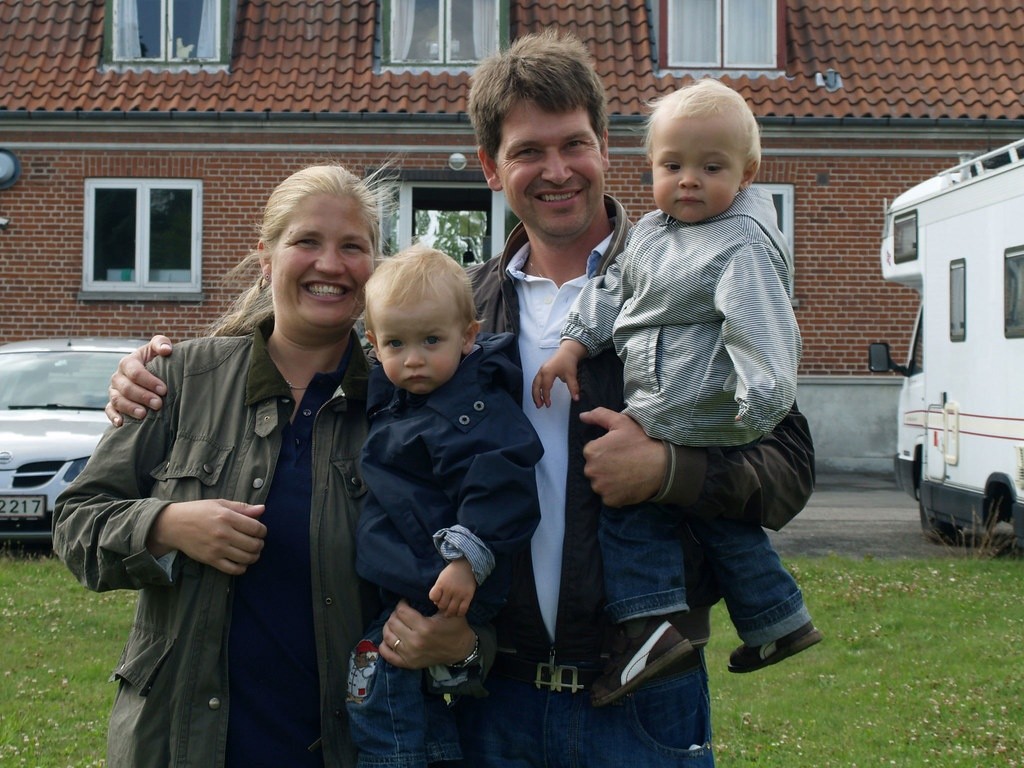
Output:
[867,133,1023,549]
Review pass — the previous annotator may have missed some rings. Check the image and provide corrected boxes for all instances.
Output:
[393,639,401,649]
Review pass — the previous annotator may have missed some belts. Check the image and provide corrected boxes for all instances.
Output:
[491,649,702,693]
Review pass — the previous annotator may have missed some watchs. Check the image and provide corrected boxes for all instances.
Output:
[447,635,480,669]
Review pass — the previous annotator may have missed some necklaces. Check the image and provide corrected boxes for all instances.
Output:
[286,380,306,391]
[529,260,543,278]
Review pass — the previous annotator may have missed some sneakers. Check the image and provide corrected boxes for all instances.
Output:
[728,620,822,673]
[591,614,694,708]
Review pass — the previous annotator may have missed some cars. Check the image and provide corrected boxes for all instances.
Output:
[0,334,150,544]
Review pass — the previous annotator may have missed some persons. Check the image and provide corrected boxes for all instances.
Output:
[52,163,493,768]
[535,76,824,705]
[105,29,817,767]
[347,243,544,768]
